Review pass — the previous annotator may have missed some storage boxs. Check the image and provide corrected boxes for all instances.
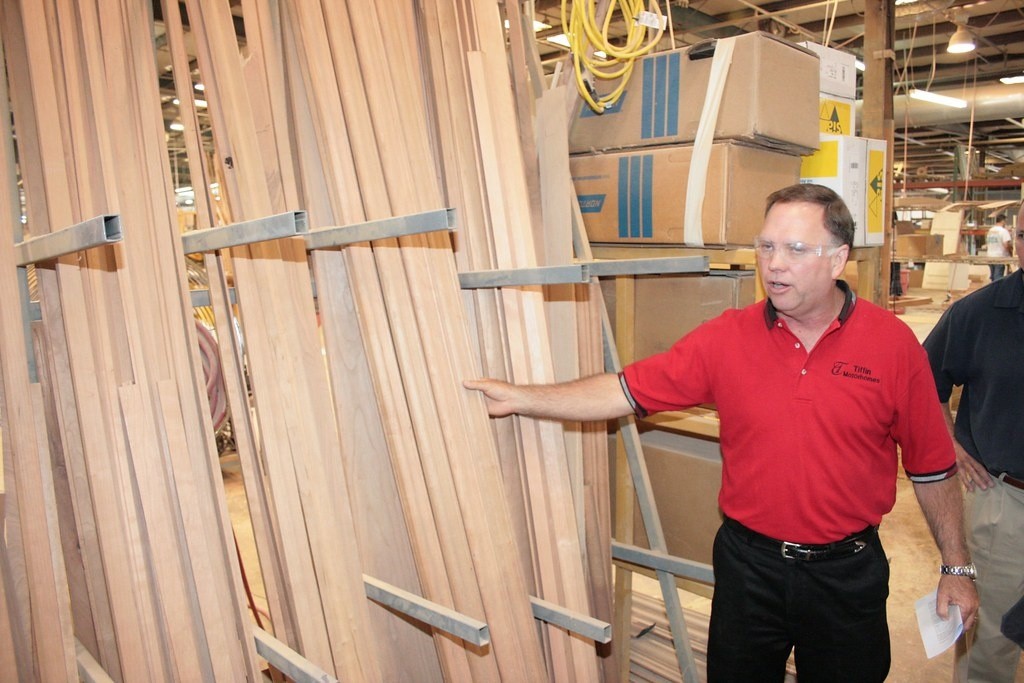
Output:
[895,221,944,260]
[798,41,889,248]
[547,30,819,601]
[908,270,924,288]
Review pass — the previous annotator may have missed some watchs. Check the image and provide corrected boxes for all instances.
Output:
[940,562,978,582]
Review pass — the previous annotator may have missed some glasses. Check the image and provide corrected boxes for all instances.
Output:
[753,238,841,263]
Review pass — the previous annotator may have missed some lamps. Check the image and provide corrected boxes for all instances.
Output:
[946,5,975,53]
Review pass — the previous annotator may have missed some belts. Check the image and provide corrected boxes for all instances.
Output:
[988,470,1024,489]
[748,537,868,562]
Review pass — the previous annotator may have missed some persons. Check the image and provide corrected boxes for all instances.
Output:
[461,182,979,683]
[920,200,1024,683]
